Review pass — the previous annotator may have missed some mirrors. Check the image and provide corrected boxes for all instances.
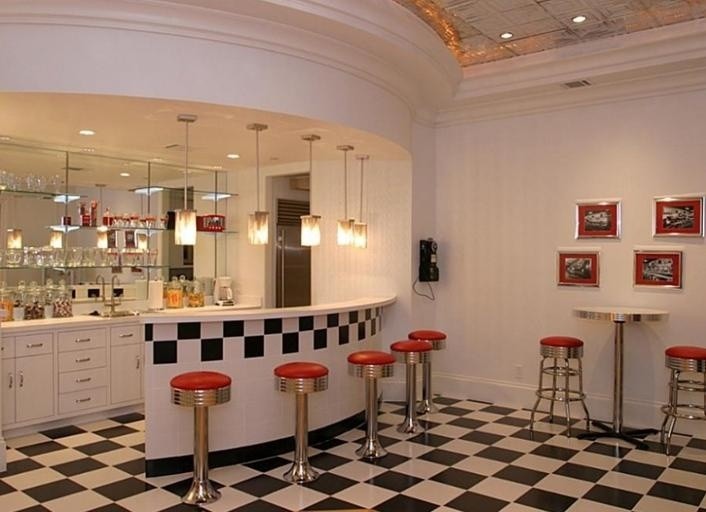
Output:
[0,138,229,307]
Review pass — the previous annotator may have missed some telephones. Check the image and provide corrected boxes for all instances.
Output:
[419,237,439,281]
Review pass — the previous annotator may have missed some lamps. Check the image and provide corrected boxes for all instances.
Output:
[336,144,356,248]
[299,134,322,246]
[6,167,148,250]
[247,124,269,246]
[174,114,198,248]
[353,154,372,248]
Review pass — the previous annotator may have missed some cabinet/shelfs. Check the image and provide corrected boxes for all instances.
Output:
[0,185,240,271]
[56,324,110,418]
[110,324,146,409]
[1,329,57,433]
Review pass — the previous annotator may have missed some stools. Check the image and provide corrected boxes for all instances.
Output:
[275,364,328,481]
[390,341,432,433]
[659,347,705,458]
[170,372,232,504]
[528,336,590,438]
[408,331,446,413]
[348,351,397,458]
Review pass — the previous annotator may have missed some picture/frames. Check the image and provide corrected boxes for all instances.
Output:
[574,199,622,240]
[632,248,683,289]
[556,248,600,287]
[651,193,705,238]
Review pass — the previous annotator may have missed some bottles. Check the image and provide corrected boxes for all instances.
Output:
[167,277,182,308]
[0,281,15,322]
[23,281,47,321]
[51,278,73,317]
[102,208,113,227]
[187,276,206,308]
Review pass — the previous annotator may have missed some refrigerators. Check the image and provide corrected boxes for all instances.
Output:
[275,197,311,307]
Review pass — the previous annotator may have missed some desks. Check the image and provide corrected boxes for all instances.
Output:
[573,307,669,453]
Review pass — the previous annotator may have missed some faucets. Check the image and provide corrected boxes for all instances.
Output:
[111,275,121,304]
[96,274,106,303]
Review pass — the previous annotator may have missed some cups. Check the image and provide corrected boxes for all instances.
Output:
[0,169,61,194]
[0,248,157,268]
[114,213,167,228]
[62,216,72,225]
[81,214,90,227]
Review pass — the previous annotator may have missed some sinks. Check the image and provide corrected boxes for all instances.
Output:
[82,310,135,318]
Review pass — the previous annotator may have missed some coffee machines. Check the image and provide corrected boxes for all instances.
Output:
[212,276,234,306]
